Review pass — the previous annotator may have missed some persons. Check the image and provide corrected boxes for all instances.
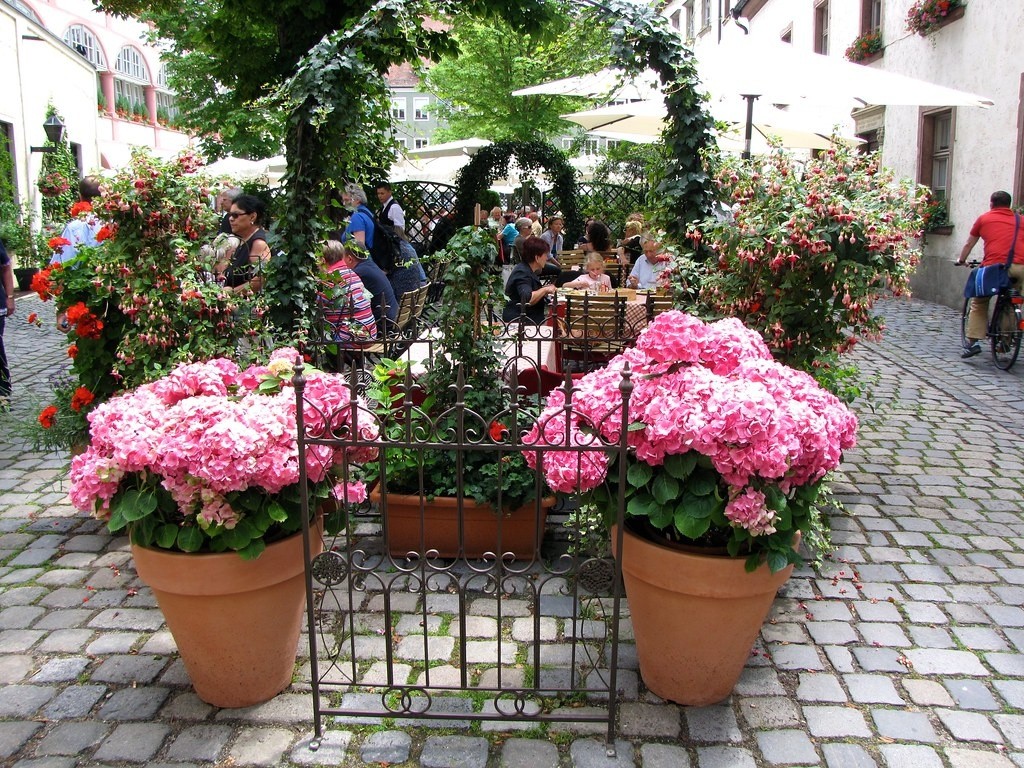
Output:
[383,225,427,304]
[339,183,374,250]
[0,239,15,402]
[376,181,405,231]
[529,212,542,237]
[217,190,238,235]
[313,240,377,373]
[502,237,557,326]
[562,252,612,290]
[178,232,240,304]
[223,194,273,359]
[513,218,561,277]
[956,190,1024,359]
[577,218,612,250]
[624,231,681,290]
[622,213,644,261]
[50,176,120,398]
[540,216,563,266]
[480,206,520,252]
[343,239,399,330]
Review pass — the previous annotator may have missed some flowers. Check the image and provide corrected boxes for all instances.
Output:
[904,0,965,50]
[0,148,947,574]
[845,29,881,62]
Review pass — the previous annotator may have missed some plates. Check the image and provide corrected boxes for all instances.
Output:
[556,287,575,292]
[635,290,656,294]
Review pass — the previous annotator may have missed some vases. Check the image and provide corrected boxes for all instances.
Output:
[128,481,801,708]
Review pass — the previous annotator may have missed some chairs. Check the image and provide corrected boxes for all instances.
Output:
[432,259,452,317]
[557,249,586,272]
[422,260,442,327]
[562,290,628,367]
[646,290,673,324]
[407,281,433,335]
[339,289,420,363]
[585,250,617,265]
[508,243,557,287]
[578,263,623,286]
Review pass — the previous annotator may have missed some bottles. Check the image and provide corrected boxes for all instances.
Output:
[595,275,611,293]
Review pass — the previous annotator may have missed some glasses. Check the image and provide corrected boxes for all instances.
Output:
[522,225,532,230]
[227,213,247,219]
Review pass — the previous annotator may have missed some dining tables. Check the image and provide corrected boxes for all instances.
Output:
[548,286,673,341]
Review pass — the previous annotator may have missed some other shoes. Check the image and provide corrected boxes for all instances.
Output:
[999,339,1011,354]
[960,345,981,359]
[1,405,15,413]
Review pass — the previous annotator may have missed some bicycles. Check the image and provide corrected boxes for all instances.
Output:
[953,260,1024,370]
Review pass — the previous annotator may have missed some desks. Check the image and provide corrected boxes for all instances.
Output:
[376,317,585,424]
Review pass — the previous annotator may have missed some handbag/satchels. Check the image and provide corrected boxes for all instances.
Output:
[964,263,1010,298]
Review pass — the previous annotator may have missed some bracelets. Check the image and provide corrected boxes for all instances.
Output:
[959,260,965,263]
[6,295,14,298]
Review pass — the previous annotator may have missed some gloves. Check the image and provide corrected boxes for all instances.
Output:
[957,259,969,268]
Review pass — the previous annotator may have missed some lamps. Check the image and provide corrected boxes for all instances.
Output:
[31,112,65,152]
[77,44,85,55]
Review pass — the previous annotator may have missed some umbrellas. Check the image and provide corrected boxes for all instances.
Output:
[187,137,647,194]
[511,31,994,155]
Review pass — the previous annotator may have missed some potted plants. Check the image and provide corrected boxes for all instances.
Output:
[97,89,222,142]
[14,199,39,290]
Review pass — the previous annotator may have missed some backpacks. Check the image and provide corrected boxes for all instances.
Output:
[355,208,398,272]
